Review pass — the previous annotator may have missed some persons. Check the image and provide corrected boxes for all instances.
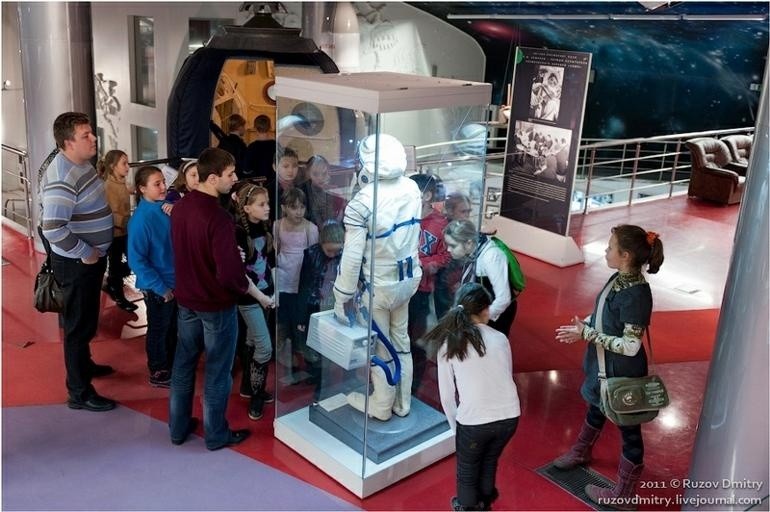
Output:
[553,226,664,511]
[440,124,487,231]
[94,149,138,313]
[508,68,570,181]
[40,111,116,412]
[416,281,521,512]
[37,144,58,257]
[442,220,516,339]
[127,166,174,389]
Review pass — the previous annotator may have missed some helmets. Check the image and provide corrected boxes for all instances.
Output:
[355,132,407,188]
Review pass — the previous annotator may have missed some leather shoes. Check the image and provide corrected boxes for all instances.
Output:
[116,296,138,312]
[67,394,116,411]
[171,417,198,445]
[92,363,113,377]
[206,429,251,451]
[100,282,117,299]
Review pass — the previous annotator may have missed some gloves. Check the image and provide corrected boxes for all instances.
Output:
[333,302,357,326]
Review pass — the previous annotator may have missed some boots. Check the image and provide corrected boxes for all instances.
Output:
[248,356,270,420]
[348,361,395,421]
[554,419,601,471]
[585,454,645,510]
[240,343,274,402]
[391,350,413,416]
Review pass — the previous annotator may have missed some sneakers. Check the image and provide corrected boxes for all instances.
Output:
[450,497,462,511]
[147,362,172,388]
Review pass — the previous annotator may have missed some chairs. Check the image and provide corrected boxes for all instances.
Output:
[718,133,753,171]
[682,136,749,210]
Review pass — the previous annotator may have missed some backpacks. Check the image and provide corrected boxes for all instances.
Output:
[468,237,525,293]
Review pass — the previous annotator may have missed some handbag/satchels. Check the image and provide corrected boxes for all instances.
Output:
[599,373,669,427]
[33,270,63,314]
